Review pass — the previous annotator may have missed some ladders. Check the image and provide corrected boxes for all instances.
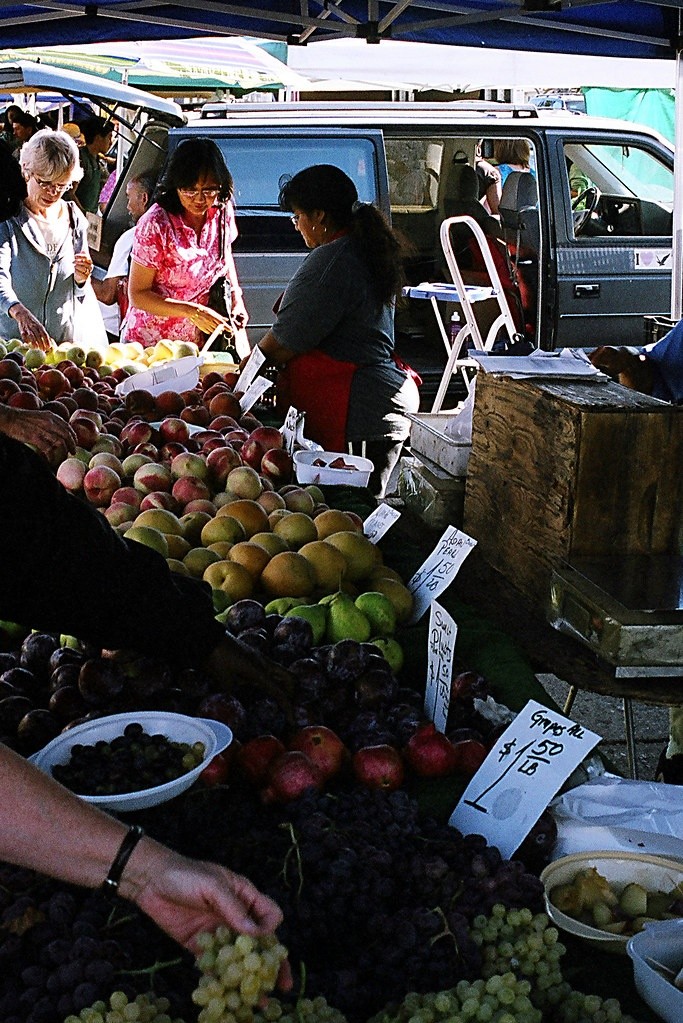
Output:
[402,214,521,415]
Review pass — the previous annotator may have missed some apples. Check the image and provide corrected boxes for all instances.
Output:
[202,725,487,800]
[0,337,412,619]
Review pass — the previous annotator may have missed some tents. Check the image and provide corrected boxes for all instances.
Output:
[281,37,676,101]
[0,37,287,181]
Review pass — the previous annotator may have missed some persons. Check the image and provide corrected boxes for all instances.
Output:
[0,403,79,461]
[385,145,424,205]
[474,139,593,211]
[0,427,216,646]
[0,741,294,1008]
[1,129,94,350]
[241,165,423,498]
[69,113,115,216]
[590,318,683,402]
[0,106,55,225]
[92,170,158,315]
[120,137,250,350]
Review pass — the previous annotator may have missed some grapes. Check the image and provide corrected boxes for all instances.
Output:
[52,722,204,797]
[0,793,630,1023]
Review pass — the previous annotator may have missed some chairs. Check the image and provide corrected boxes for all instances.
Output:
[443,165,501,266]
[498,172,540,262]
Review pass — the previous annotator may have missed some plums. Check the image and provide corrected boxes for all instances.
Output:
[0,599,490,745]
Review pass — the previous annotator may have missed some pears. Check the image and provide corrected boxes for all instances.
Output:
[330,592,370,643]
[357,591,398,636]
[265,597,309,615]
[56,634,81,652]
[286,604,326,646]
[318,595,342,605]
[372,639,403,672]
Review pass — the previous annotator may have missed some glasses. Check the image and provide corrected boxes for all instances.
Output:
[177,187,221,199]
[30,172,74,192]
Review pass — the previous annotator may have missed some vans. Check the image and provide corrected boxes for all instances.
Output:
[15,58,675,429]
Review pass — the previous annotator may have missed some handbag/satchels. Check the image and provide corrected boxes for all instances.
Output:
[205,276,242,365]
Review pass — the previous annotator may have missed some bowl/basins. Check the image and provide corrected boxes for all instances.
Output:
[538,850,683,952]
[292,449,375,488]
[114,355,204,399]
[625,918,683,1023]
[22,710,233,813]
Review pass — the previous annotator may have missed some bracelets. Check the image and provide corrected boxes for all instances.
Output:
[102,825,145,898]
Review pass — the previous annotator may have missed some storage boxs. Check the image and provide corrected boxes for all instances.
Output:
[405,410,471,478]
[461,361,682,606]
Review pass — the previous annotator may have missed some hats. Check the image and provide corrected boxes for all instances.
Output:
[55,123,86,147]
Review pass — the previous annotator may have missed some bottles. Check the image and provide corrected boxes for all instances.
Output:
[444,311,468,360]
